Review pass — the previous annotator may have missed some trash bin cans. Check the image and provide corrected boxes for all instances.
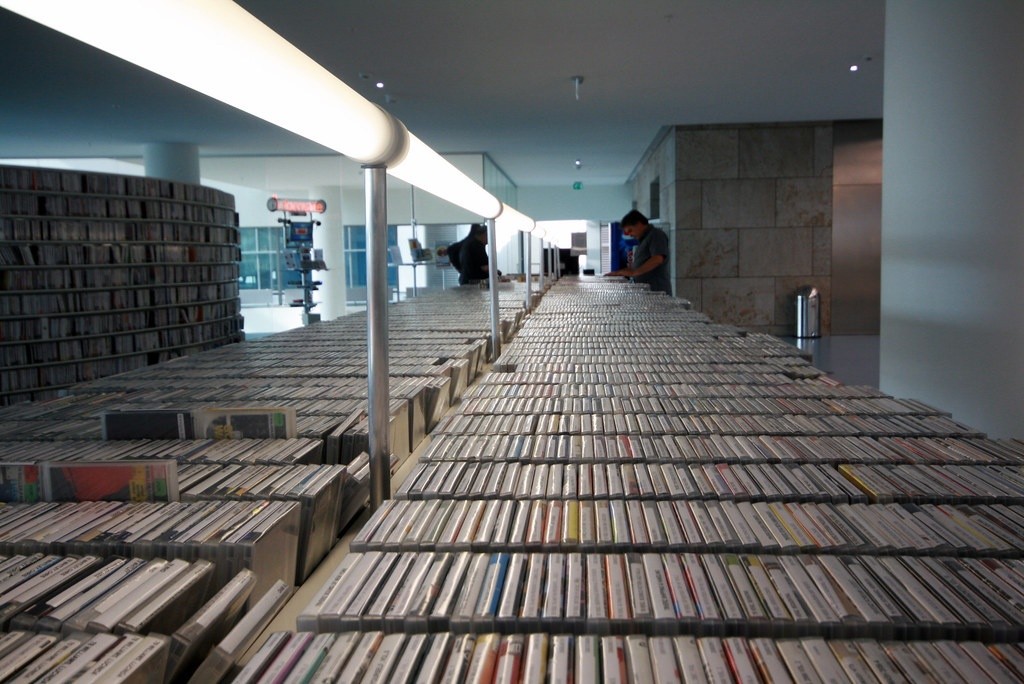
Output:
[794,283,821,338]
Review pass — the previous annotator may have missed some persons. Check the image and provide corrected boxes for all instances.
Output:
[604,209,672,294]
[446,224,501,284]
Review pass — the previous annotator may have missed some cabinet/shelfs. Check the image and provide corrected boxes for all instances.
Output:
[0,188,242,395]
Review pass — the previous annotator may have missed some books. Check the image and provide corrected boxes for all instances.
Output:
[0,163,244,409]
